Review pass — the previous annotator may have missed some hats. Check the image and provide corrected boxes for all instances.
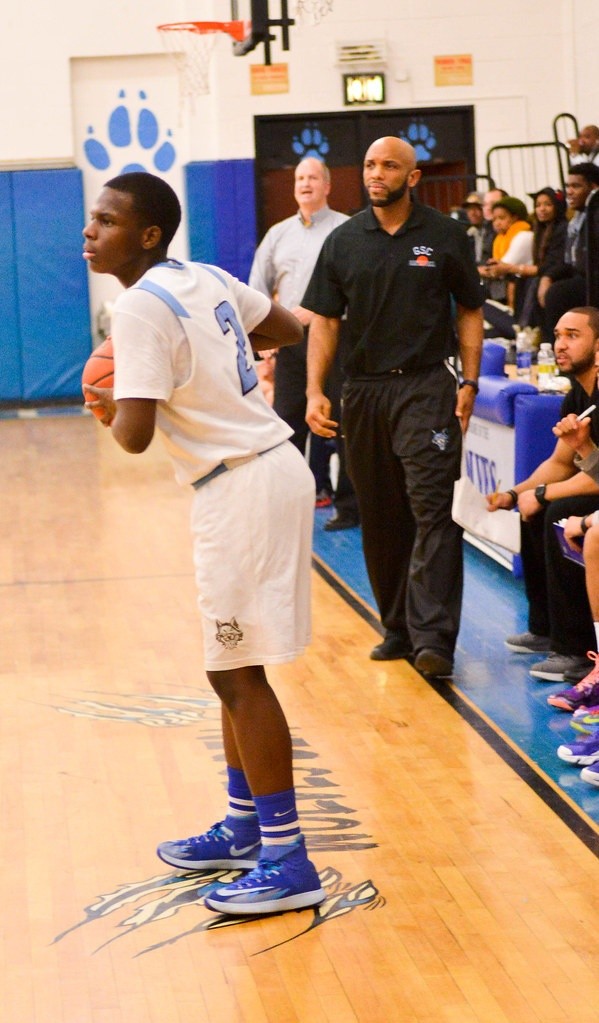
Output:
[462,194,481,207]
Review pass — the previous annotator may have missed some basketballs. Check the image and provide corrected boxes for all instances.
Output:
[81,336,118,427]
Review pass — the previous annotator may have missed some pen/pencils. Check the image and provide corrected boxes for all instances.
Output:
[492,479,501,502]
[553,404,597,439]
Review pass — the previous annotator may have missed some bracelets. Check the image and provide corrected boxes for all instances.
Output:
[498,489,518,510]
[581,515,589,533]
[515,263,525,277]
[458,380,479,395]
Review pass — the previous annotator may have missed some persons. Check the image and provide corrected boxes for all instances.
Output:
[81,173,316,914]
[299,136,488,676]
[547,350,599,710]
[248,157,363,532]
[556,705,599,786]
[485,306,599,682]
[441,124,599,344]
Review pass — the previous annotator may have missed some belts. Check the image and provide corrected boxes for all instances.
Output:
[191,442,283,490]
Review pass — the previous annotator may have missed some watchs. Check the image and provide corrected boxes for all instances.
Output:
[534,484,552,507]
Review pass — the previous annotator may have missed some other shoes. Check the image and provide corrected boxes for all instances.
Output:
[414,648,453,677]
[371,640,412,660]
[315,489,332,507]
[324,511,360,531]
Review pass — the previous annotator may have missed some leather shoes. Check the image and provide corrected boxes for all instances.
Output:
[563,665,595,686]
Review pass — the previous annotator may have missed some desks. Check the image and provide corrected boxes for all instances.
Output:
[448,337,566,580]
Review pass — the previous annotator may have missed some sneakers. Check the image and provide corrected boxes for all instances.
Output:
[580,762,599,786]
[557,731,599,765]
[204,834,327,914]
[528,651,589,681]
[547,651,599,711]
[573,706,599,717]
[157,814,260,869]
[504,631,550,653]
[569,714,599,734]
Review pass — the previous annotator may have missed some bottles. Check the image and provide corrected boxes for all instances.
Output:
[536,343,557,390]
[516,332,533,378]
